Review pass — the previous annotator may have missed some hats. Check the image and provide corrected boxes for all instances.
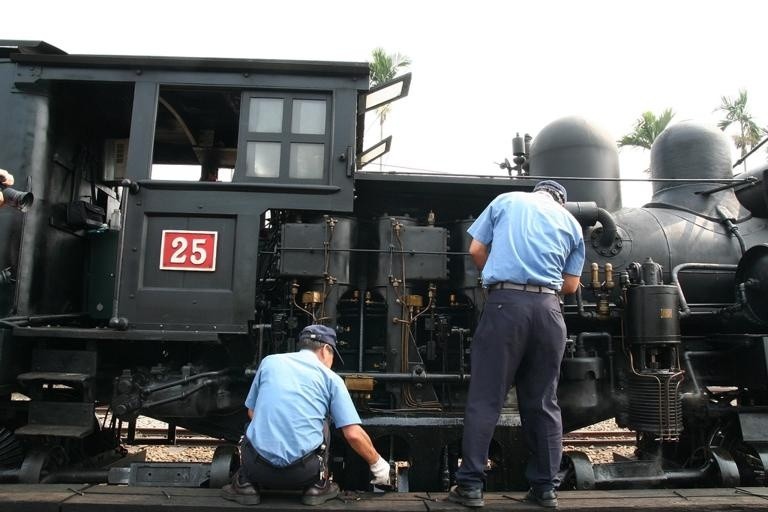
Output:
[535,179,567,204]
[298,325,344,364]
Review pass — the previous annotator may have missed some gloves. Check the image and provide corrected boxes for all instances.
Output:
[370,454,391,485]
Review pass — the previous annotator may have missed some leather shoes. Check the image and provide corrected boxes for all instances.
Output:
[448,484,485,507]
[302,481,340,506]
[220,473,261,505]
[526,488,559,506]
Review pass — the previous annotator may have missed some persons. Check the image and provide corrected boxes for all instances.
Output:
[445,179,587,508]
[220,324,389,506]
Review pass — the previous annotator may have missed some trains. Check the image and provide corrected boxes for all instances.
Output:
[0,40,767,494]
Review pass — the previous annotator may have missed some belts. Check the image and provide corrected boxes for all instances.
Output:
[491,281,557,295]
[242,435,327,470]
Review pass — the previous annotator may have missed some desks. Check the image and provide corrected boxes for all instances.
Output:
[85,230,119,319]
